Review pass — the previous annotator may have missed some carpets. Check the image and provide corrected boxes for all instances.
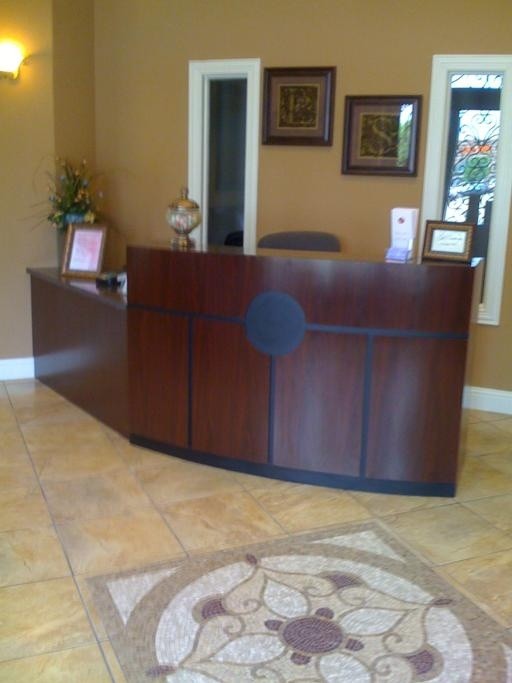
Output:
[84,517,512,683]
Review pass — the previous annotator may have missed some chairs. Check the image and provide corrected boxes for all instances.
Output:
[257,230,341,252]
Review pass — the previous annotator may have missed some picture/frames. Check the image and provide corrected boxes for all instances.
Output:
[60,220,110,280]
[341,94,422,176]
[422,220,476,262]
[262,63,336,145]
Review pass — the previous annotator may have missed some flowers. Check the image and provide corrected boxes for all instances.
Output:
[45,151,101,225]
